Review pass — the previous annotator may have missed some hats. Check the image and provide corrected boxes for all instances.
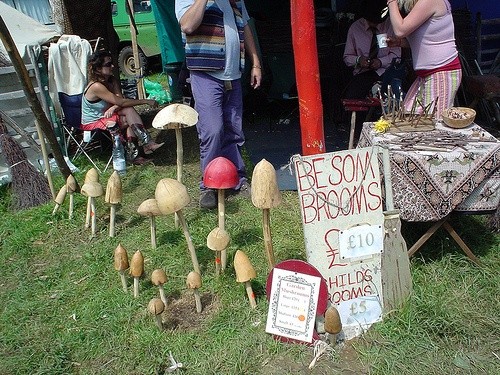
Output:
[358,0,389,22]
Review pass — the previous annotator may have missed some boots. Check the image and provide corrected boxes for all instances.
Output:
[125,136,154,165]
[131,123,165,154]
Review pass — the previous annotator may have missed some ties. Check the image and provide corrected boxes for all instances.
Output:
[369,27,379,59]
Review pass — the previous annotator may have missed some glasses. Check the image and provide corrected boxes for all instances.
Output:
[100,60,114,67]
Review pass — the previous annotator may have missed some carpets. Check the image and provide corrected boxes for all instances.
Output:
[242,101,345,191]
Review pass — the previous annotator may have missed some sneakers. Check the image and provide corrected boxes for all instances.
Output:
[200,188,218,209]
[231,181,251,196]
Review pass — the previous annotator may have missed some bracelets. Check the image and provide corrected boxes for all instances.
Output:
[387,0,396,5]
[251,66,261,69]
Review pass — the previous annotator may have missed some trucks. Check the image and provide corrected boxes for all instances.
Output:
[108,0,162,79]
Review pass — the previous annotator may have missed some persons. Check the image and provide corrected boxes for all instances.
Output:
[174,0,262,208]
[343,0,463,122]
[79,51,165,165]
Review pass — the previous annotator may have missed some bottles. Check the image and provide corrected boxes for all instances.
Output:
[382,209,413,317]
[112,136,126,176]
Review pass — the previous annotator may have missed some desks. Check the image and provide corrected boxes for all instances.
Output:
[340,97,381,149]
[359,121,499,263]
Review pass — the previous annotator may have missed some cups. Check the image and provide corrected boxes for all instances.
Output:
[376,34,388,48]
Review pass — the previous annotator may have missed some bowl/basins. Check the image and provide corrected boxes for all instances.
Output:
[441,107,476,128]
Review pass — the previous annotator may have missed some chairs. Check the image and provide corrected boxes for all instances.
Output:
[58,92,116,172]
[262,51,300,131]
